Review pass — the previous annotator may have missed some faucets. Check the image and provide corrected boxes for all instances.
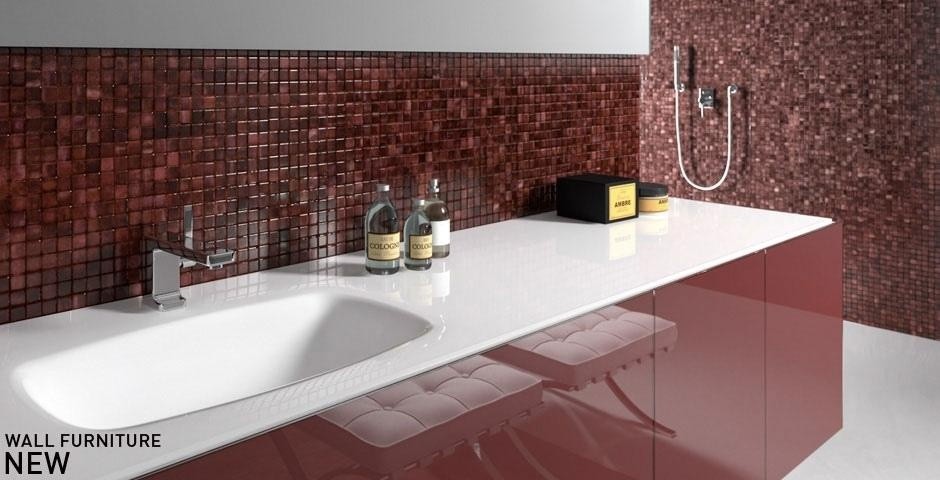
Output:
[142,229,236,312]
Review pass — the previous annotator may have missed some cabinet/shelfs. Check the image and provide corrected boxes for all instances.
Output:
[131,219,843,480]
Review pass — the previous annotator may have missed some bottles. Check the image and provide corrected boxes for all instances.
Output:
[364,177,452,272]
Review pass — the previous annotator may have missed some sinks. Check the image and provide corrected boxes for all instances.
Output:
[17,292,436,430]
